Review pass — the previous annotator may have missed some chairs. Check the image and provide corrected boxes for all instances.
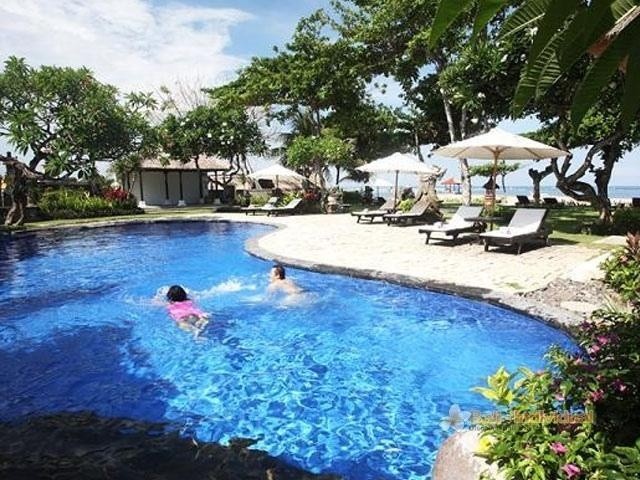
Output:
[350,199,553,256]
[240,197,302,216]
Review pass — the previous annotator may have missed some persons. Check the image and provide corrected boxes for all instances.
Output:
[164,284,210,339]
[264,263,309,305]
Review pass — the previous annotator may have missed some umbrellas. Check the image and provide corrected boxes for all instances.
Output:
[436,126,571,231]
[353,152,438,212]
[245,164,307,188]
